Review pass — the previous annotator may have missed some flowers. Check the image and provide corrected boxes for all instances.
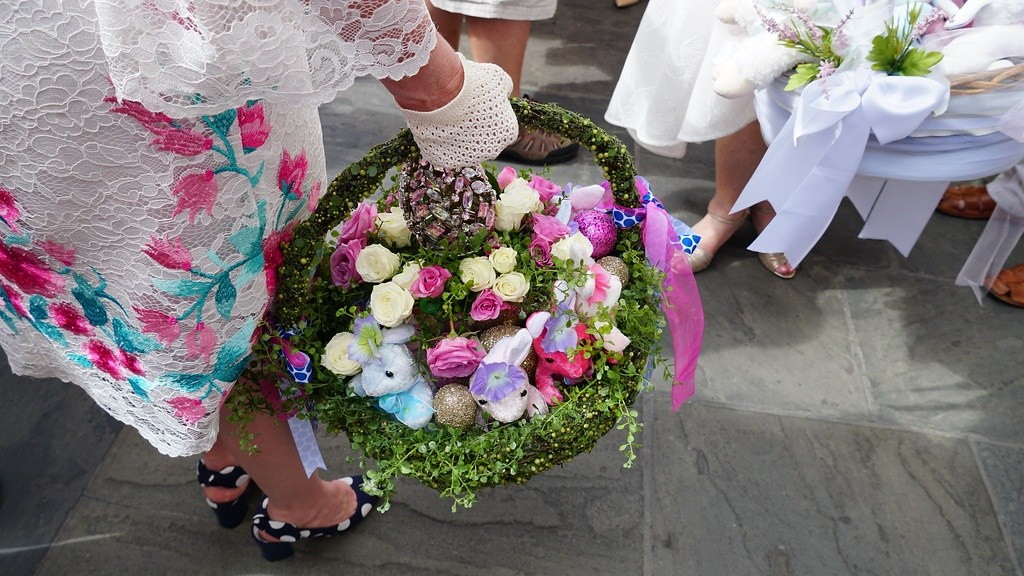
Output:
[311,160,633,405]
[751,0,944,104]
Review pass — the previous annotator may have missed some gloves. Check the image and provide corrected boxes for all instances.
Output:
[390,51,518,168]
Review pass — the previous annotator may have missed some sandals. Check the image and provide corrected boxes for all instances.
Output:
[504,120,580,164]
[669,205,750,273]
[754,205,796,279]
[937,185,1022,218]
[981,266,1024,305]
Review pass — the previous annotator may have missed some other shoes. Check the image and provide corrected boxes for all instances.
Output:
[615,0,638,7]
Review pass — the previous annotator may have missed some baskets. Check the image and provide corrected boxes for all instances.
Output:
[226,100,705,515]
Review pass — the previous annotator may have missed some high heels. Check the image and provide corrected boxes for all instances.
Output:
[249,476,385,562]
[195,458,260,529]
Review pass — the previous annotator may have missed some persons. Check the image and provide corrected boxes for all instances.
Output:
[602,0,1024,308]
[0,0,581,562]
